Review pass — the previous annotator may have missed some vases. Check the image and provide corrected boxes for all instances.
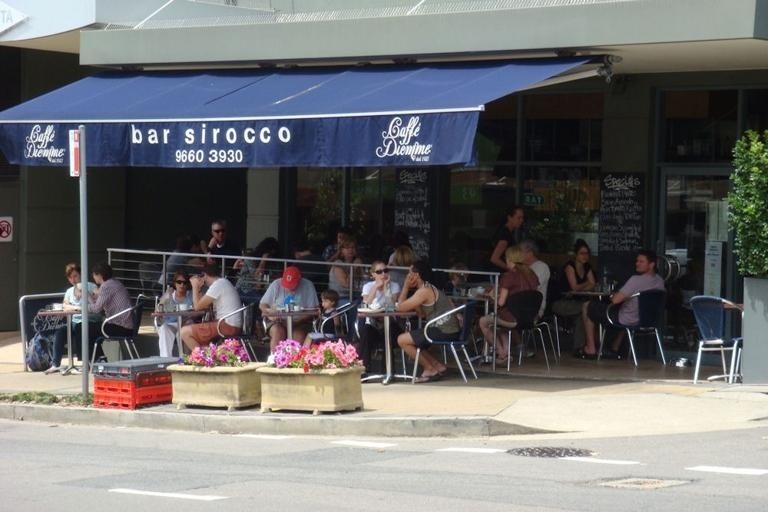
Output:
[166,362,366,415]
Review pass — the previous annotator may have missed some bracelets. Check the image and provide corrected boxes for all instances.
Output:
[74,307,78,311]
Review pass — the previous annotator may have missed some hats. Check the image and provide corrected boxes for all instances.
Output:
[280,264,303,290]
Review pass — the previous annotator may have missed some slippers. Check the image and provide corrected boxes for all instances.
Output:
[575,345,598,360]
[602,346,620,360]
[413,366,451,383]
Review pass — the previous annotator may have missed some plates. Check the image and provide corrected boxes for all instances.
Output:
[176,308,193,312]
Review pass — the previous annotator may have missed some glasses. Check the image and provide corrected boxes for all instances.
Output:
[373,267,390,274]
[212,229,226,234]
[174,279,188,285]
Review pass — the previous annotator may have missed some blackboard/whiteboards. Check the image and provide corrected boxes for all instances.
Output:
[598,171,643,282]
[394,166,432,261]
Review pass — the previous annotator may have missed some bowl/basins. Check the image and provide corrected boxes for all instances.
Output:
[477,288,485,294]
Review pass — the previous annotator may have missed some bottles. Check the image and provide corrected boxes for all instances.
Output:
[595,265,616,294]
[158,304,164,313]
[357,282,396,313]
[270,297,319,312]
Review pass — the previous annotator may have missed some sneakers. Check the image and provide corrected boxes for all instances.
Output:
[516,349,535,357]
[43,365,61,374]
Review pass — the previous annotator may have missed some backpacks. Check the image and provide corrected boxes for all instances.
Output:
[26,331,53,373]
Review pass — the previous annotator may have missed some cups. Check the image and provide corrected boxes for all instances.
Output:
[179,304,189,311]
[45,303,64,312]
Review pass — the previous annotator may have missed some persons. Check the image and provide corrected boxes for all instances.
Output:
[136,202,551,384]
[44,262,102,374]
[570,249,665,360]
[550,239,599,318]
[65,264,134,363]
